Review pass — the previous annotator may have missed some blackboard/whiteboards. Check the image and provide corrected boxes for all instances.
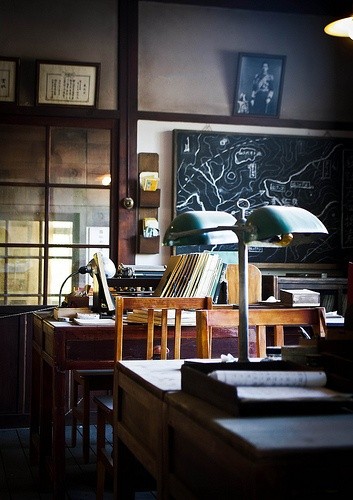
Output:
[174,130,353,268]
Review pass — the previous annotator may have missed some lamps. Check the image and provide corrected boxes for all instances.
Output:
[159,198,330,360]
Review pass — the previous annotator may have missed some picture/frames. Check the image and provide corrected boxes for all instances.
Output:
[233,52,287,119]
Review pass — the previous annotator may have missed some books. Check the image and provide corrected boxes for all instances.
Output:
[126,309,196,327]
[151,253,228,304]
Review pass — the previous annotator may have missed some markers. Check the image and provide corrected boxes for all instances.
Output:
[73,286,75,292]
[77,286,79,290]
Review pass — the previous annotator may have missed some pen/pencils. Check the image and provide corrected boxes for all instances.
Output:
[297,325,311,339]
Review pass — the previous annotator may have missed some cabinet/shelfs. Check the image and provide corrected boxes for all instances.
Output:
[138,152,160,254]
[261,275,348,347]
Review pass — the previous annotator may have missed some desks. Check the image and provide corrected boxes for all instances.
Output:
[163,390,353,500]
[28,313,343,500]
[113,357,265,500]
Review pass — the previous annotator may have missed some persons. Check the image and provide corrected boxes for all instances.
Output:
[249,62,274,115]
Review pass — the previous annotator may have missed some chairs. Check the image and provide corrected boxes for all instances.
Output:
[193,306,326,359]
[93,296,214,499]
[69,367,113,455]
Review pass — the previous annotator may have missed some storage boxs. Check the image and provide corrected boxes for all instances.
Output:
[54,307,90,320]
[179,361,353,419]
[66,295,89,307]
